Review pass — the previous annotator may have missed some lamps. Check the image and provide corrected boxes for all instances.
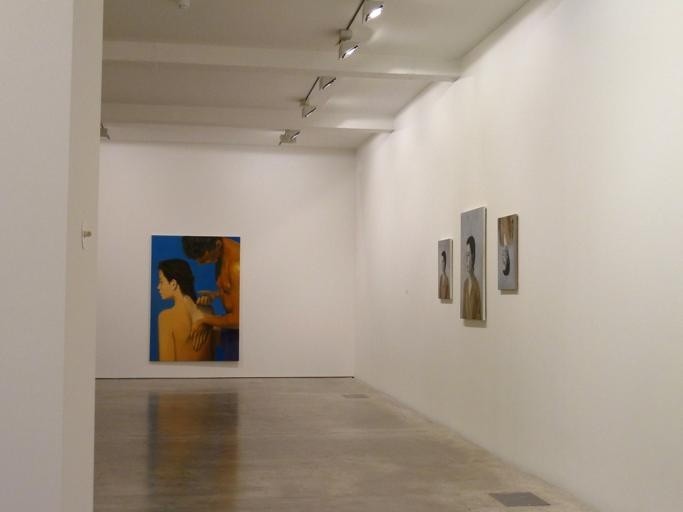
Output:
[101,122,111,142]
[279,0,384,144]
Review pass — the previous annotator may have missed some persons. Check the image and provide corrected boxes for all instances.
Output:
[157,236,240,361]
[438,251,450,299]
[461,236,482,320]
[498,217,517,276]
